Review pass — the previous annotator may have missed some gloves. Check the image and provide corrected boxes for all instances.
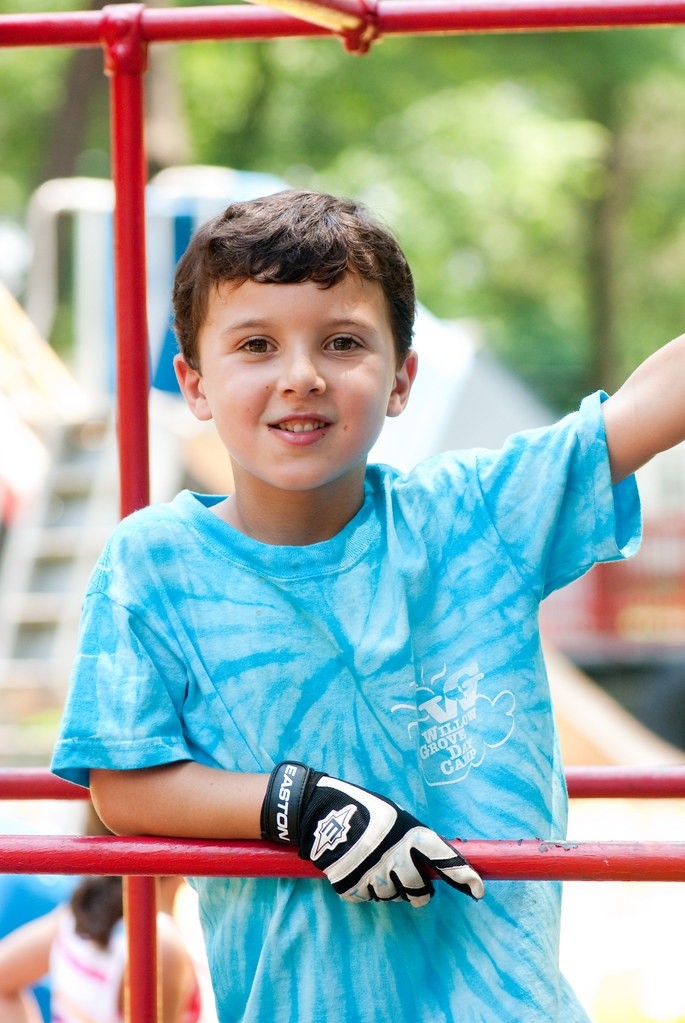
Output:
[260,761,486,910]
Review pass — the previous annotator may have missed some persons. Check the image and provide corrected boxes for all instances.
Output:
[53,190,685,1023]
[2,798,208,1023]
